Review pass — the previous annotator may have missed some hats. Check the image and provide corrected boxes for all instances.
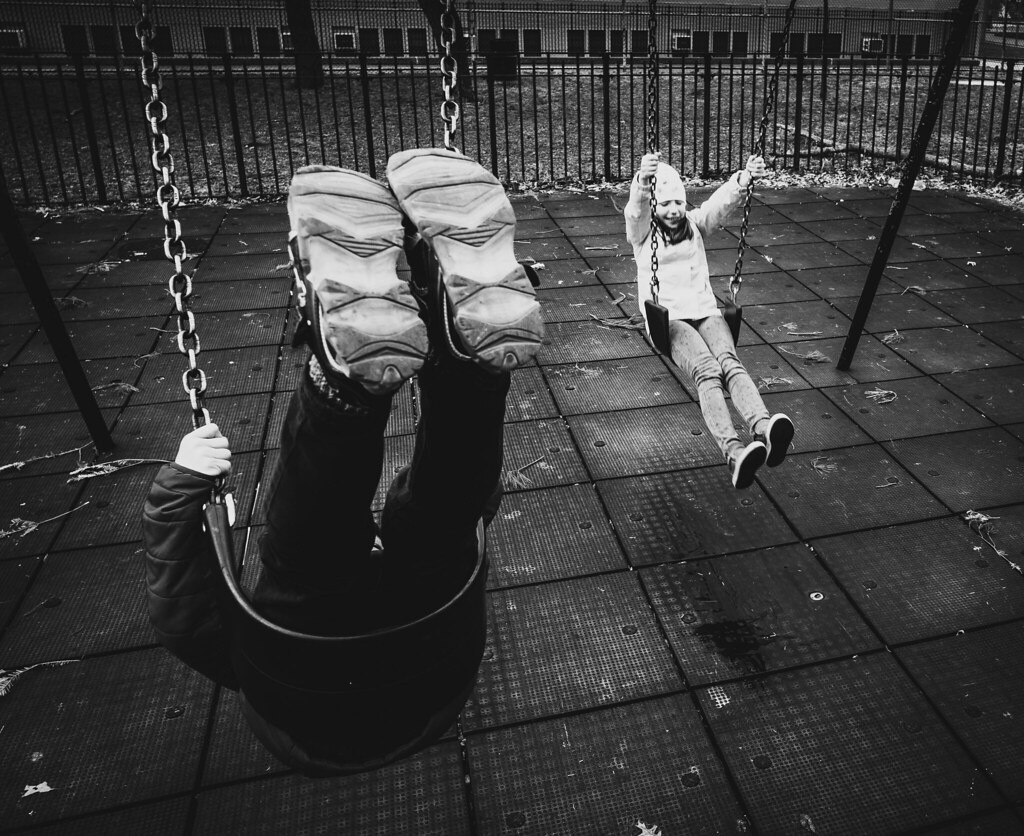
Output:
[651,162,686,205]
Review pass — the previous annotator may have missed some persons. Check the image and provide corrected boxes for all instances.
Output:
[625,152,795,491]
[142,145,545,780]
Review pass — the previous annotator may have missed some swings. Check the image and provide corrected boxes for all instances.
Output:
[135,0,488,696]
[644,0,796,366]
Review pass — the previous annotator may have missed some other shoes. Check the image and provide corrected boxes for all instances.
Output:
[727,441,766,490]
[763,413,795,467]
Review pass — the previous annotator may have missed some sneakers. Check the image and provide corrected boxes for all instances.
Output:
[387,147,544,372]
[277,165,429,403]
